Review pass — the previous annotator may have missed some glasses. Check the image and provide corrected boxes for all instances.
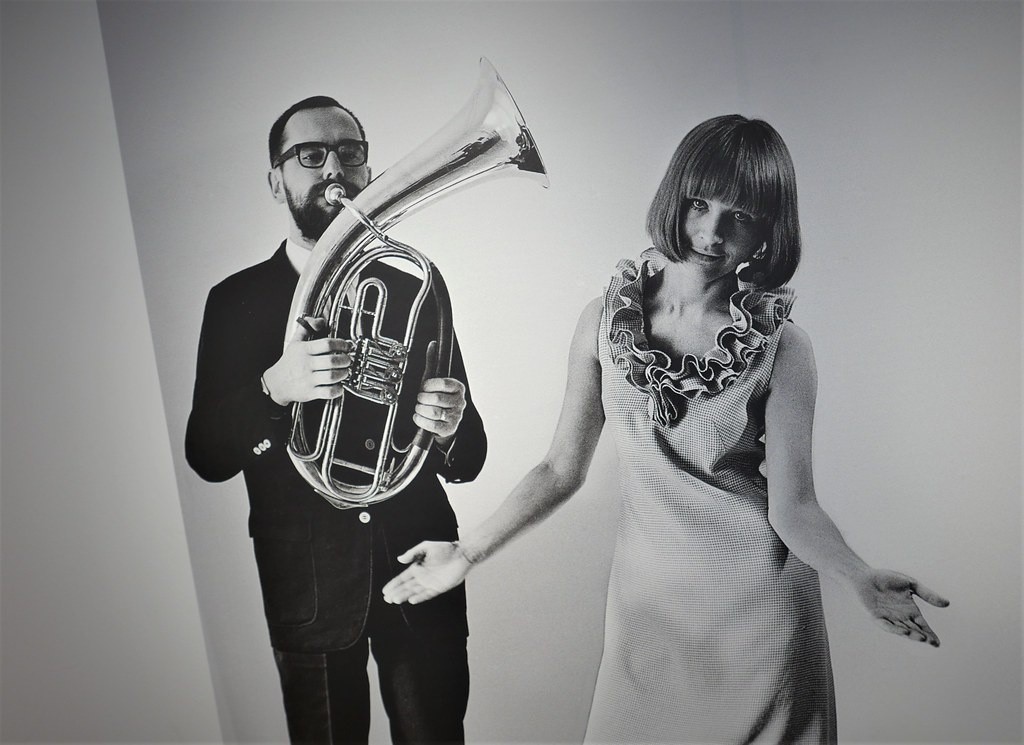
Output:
[272,138,369,168]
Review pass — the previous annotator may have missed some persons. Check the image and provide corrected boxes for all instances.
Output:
[381,114,949,745]
[184,95,489,744]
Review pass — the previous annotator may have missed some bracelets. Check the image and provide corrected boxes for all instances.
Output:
[253,374,289,418]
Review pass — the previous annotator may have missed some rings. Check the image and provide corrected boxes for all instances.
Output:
[441,408,447,421]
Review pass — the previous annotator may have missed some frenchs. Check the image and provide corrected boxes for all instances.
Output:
[280,51,553,513]
